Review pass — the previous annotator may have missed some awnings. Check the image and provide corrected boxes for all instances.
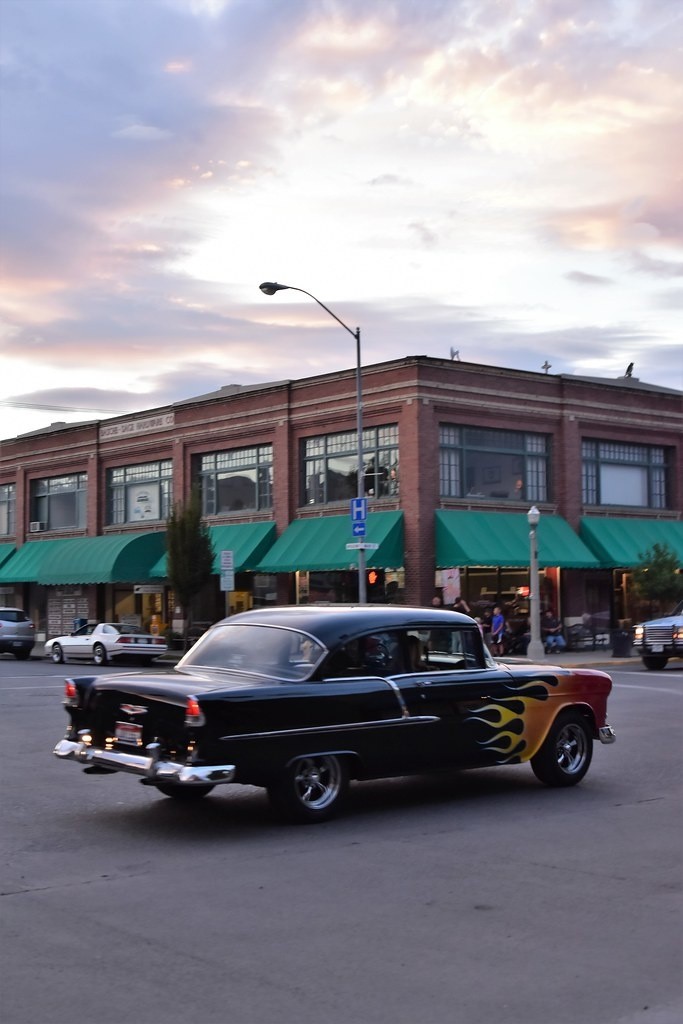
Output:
[579,515,683,569]
[0,518,276,585]
[434,509,600,569]
[253,510,405,574]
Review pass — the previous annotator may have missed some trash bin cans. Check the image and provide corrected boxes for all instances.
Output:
[611,629,634,658]
[73,618,88,636]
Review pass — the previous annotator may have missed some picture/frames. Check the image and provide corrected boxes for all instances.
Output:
[511,456,523,474]
[481,465,502,485]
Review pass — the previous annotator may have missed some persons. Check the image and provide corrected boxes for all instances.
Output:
[426,596,567,657]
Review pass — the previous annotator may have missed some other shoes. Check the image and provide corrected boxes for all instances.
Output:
[547,650,551,653]
[556,650,560,654]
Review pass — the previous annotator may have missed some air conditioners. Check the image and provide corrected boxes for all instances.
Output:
[30,522,46,532]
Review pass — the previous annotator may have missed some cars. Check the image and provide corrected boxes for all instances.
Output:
[632,600,683,671]
[43,621,169,668]
[53,596,618,824]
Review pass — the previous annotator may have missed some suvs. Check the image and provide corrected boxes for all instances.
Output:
[0,606,37,661]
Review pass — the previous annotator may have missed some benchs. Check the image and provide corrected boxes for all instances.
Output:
[566,623,606,652]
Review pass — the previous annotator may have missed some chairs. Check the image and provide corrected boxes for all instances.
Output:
[355,634,419,677]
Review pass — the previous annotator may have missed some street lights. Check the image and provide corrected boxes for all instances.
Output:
[259,281,367,604]
[525,506,546,660]
